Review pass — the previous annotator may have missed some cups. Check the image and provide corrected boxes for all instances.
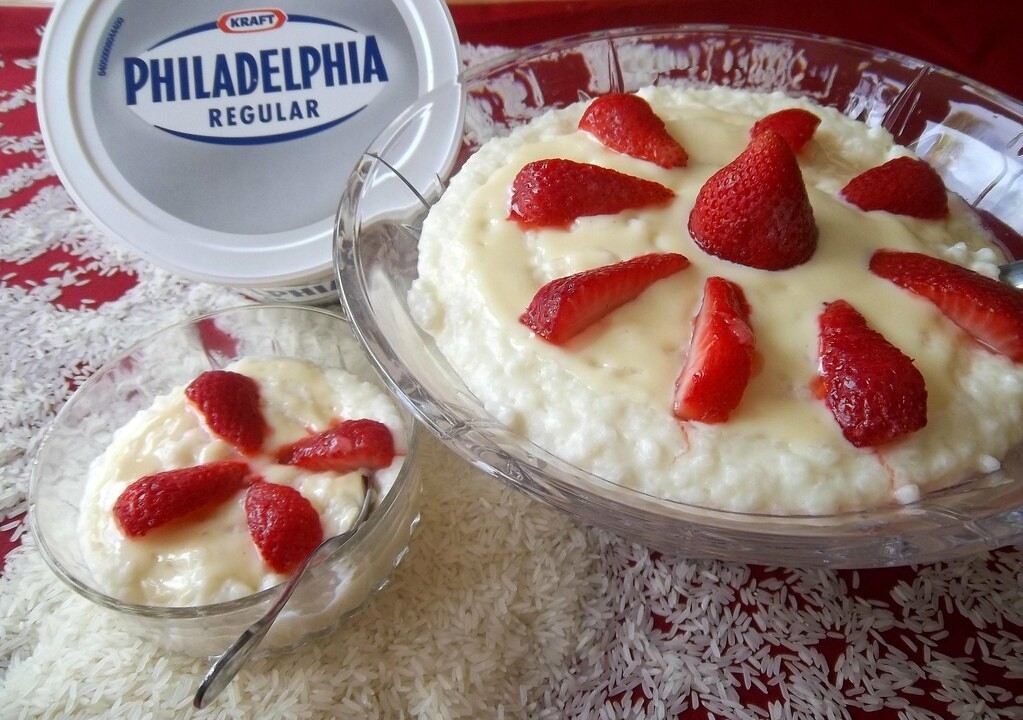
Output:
[33,0,464,308]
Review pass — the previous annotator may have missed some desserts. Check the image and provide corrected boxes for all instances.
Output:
[73,351,417,661]
[401,84,1023,522]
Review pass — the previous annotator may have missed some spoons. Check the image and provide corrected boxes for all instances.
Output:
[192,473,374,710]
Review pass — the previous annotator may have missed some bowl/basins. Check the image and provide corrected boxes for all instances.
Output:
[332,25,1023,569]
[27,304,424,660]
